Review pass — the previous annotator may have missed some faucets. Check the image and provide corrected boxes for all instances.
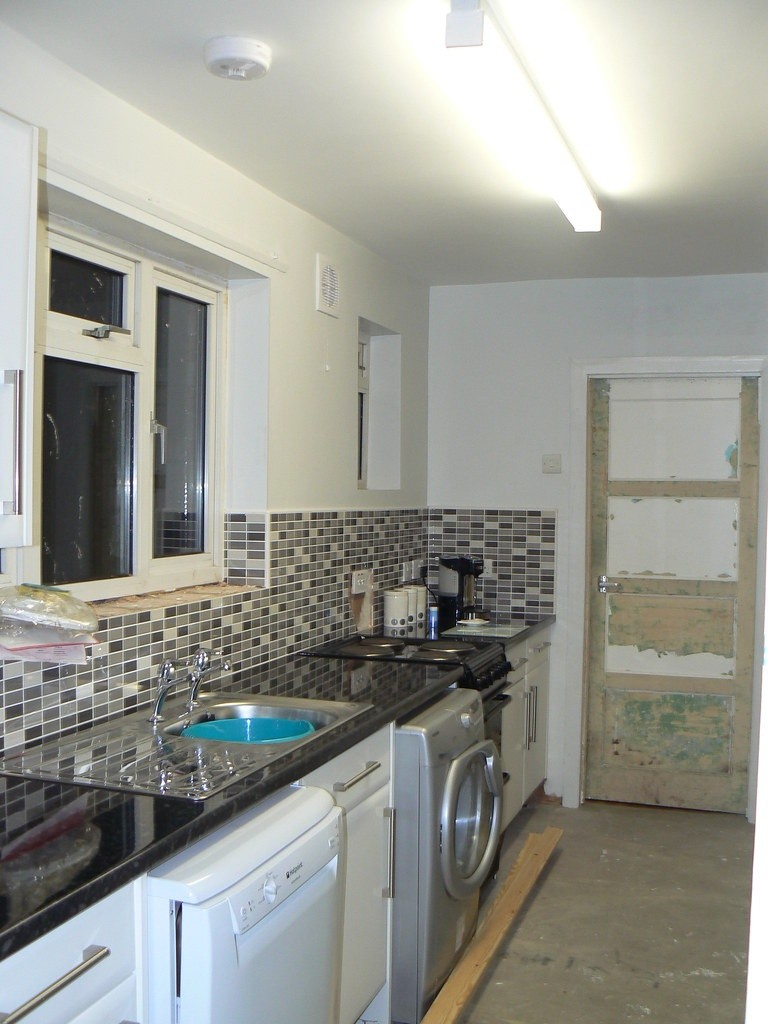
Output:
[183,648,232,708]
[148,658,200,722]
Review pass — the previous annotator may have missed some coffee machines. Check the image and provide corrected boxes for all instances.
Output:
[437,553,491,623]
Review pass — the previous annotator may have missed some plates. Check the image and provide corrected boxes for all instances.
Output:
[457,620,490,627]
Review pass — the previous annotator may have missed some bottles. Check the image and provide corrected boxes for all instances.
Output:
[382,584,427,628]
[429,607,439,628]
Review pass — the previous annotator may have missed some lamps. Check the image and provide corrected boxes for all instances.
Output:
[440,0,605,237]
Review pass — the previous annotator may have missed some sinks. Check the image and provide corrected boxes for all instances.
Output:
[163,702,352,746]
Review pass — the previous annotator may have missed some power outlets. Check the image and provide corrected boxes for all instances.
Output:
[419,560,424,578]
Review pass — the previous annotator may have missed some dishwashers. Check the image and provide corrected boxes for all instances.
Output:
[144,783,348,1024]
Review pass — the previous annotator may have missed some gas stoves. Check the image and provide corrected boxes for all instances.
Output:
[299,634,515,688]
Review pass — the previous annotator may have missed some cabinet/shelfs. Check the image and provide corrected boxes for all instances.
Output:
[0,109,38,548]
[502,622,551,832]
[299,719,397,1024]
[0,873,142,1024]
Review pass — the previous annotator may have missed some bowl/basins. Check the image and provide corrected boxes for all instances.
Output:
[181,716,315,744]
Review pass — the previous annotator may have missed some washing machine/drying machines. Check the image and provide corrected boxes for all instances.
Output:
[393,687,504,1024]
